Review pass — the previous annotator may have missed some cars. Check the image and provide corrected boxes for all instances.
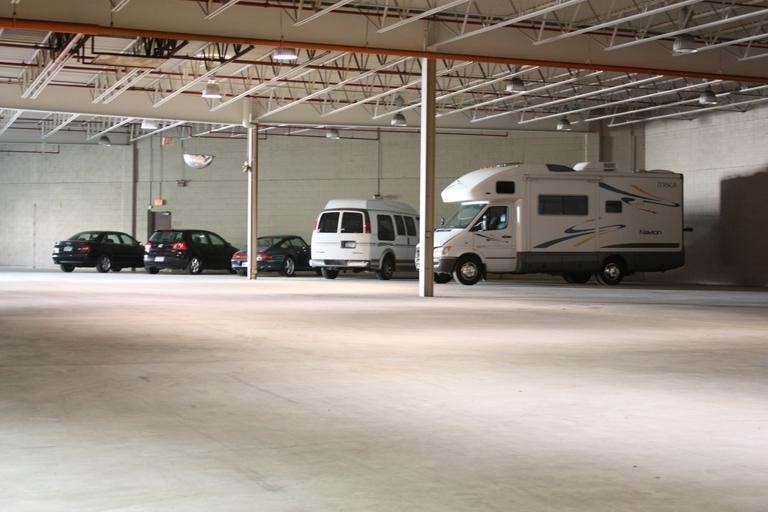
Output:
[52,231,146,273]
[142,228,240,275]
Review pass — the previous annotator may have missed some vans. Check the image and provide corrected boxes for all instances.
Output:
[309,198,421,281]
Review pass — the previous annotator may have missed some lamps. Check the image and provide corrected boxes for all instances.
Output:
[672,34,697,53]
[505,64,526,92]
[272,47,298,59]
[556,114,571,131]
[98,131,111,146]
[202,74,222,98]
[390,112,407,126]
[326,130,340,140]
[699,85,718,105]
[141,119,161,130]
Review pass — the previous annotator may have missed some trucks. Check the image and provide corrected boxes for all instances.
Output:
[415,157,688,287]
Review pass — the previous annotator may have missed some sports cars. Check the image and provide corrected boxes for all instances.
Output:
[232,233,322,278]
